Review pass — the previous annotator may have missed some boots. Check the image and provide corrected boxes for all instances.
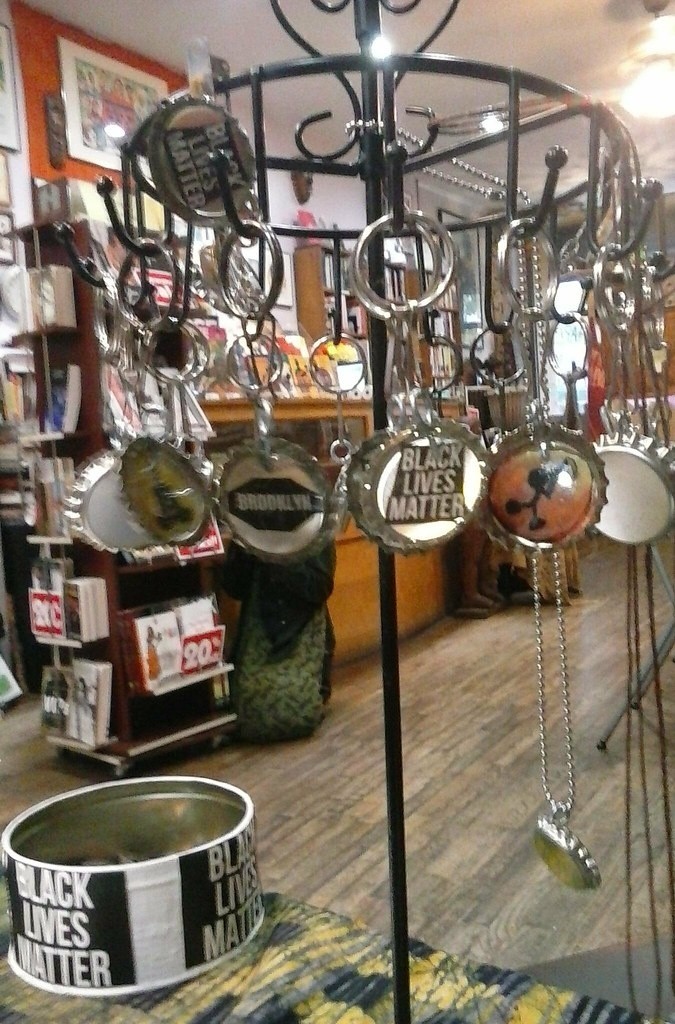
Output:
[455,528,504,606]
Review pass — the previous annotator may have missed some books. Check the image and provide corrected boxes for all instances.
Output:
[41,659,112,747]
[30,556,110,642]
[327,296,366,340]
[426,310,480,433]
[323,252,458,307]
[0,347,81,435]
[29,261,76,331]
[121,510,225,564]
[0,449,75,539]
[134,593,226,693]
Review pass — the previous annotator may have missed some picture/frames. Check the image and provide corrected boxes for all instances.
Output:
[0,24,22,267]
[56,34,169,173]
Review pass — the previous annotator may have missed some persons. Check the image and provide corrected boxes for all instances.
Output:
[147,626,162,680]
[457,417,505,608]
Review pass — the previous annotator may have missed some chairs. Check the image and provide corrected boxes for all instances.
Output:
[0,867,675,1024]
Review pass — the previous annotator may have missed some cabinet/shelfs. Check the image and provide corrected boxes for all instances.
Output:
[19,217,235,763]
[293,244,464,396]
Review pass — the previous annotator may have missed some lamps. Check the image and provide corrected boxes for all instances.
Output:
[617,0,675,123]
